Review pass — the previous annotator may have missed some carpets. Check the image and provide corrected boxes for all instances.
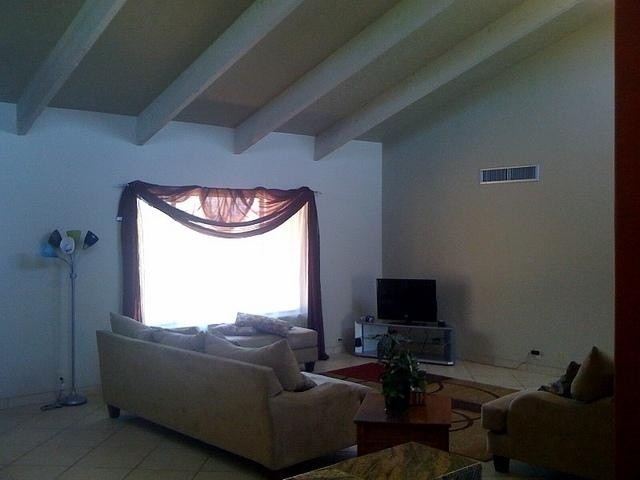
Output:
[317,361,520,461]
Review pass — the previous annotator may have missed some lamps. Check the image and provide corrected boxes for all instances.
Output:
[38,229,99,406]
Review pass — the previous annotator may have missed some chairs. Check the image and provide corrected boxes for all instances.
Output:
[481,387,614,480]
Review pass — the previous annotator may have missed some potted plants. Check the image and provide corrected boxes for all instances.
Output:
[371,333,429,414]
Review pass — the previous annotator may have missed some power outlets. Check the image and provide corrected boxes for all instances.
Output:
[531,349,542,360]
[57,376,65,387]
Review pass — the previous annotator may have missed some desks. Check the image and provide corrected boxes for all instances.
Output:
[281,442,482,480]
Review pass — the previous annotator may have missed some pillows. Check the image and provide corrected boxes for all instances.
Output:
[570,346,614,403]
[538,362,581,399]
[110,312,205,353]
[205,333,317,391]
[208,323,258,335]
[236,312,288,337]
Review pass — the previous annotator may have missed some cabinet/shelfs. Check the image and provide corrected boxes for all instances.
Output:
[355,320,455,366]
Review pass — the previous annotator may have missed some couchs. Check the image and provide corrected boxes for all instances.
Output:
[96,330,375,472]
[150,327,318,372]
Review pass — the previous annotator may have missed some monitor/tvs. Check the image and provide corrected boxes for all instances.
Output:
[376,277,438,324]
